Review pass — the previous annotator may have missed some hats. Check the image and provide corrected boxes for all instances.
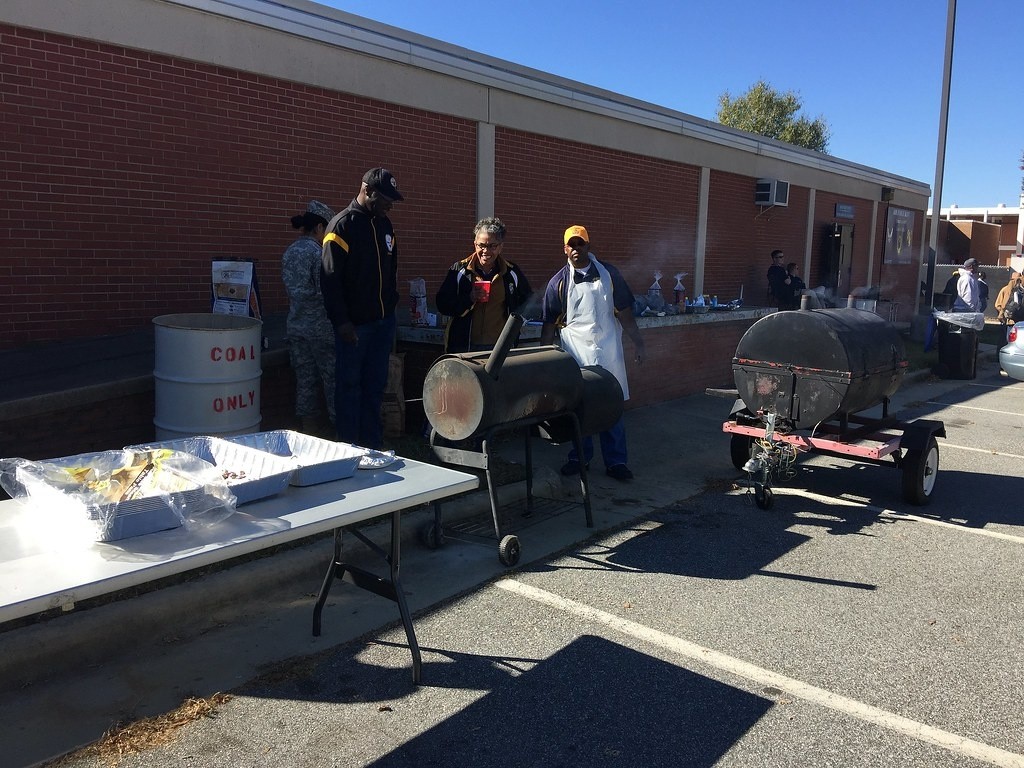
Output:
[363,166,405,202]
[1010,272,1021,280]
[563,225,589,245]
[306,200,335,224]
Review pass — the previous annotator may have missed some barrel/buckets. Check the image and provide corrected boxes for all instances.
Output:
[152,313,264,441]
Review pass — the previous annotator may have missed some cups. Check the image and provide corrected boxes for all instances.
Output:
[475,281,490,302]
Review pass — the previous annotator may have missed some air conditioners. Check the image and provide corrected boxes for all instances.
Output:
[755,179,790,206]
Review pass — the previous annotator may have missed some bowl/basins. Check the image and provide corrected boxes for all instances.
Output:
[686,306,708,313]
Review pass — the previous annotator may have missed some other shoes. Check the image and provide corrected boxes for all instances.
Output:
[561,460,589,476]
[606,464,634,479]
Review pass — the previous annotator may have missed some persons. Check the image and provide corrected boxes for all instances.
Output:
[281,200,336,444]
[766,250,807,311]
[943,258,989,314]
[429,216,531,462]
[540,225,645,485]
[995,268,1024,326]
[321,167,405,451]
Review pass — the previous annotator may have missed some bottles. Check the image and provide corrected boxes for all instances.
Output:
[713,295,718,307]
[685,297,689,305]
[703,294,710,305]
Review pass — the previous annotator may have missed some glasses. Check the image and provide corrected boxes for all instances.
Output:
[475,242,500,250]
[775,256,784,259]
[795,268,799,270]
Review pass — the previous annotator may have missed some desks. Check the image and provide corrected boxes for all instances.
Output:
[394,304,779,433]
[0,429,480,684]
